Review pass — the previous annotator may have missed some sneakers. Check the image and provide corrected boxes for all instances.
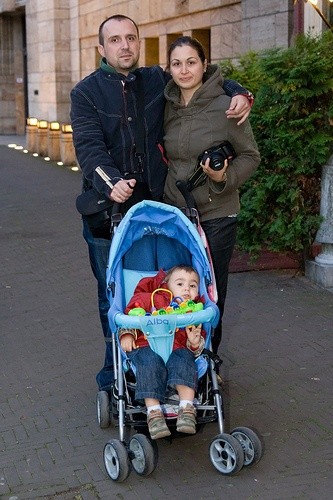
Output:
[176,403,197,434]
[147,409,171,439]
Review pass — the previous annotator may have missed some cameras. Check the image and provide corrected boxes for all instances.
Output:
[198,141,236,170]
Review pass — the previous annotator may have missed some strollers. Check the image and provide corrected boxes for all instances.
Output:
[95,180,263,483]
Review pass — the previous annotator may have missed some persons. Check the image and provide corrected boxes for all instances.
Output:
[119,263,206,439]
[68,15,254,421]
[165,36,261,355]
